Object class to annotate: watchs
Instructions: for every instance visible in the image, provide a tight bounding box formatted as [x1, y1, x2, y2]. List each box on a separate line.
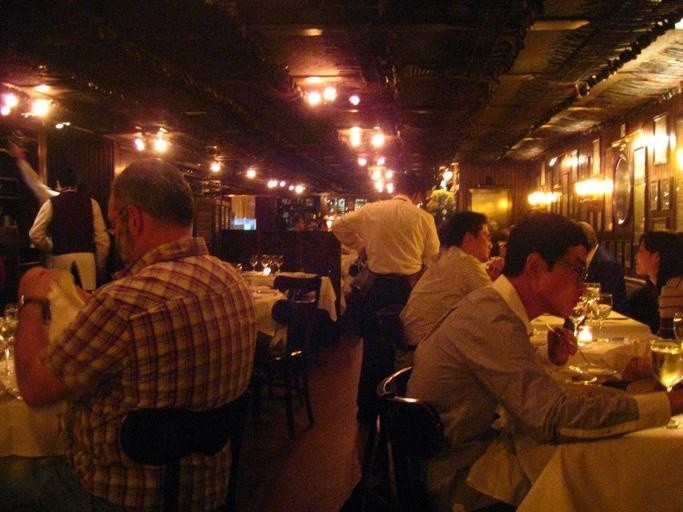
[15, 293, 46, 314]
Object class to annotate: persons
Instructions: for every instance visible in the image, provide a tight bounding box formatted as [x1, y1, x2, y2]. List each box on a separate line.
[28, 156, 110, 294]
[14, 156, 258, 511]
[332, 170, 442, 426]
[6, 139, 60, 204]
[400, 212, 683, 511]
[397, 207, 504, 379]
[574, 218, 627, 314]
[287, 210, 308, 231]
[622, 231, 683, 334]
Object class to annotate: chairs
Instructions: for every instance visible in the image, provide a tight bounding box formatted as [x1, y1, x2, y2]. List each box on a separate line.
[367, 304, 418, 454]
[121, 389, 251, 512]
[376, 364, 445, 509]
[254, 294, 320, 441]
[258, 275, 338, 408]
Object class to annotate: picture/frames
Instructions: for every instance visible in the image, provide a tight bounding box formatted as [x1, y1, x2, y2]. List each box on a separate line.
[540, 110, 674, 269]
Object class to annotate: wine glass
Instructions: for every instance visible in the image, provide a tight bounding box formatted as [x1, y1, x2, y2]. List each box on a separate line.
[557, 371, 597, 385]
[0, 304, 24, 377]
[647, 309, 682, 428]
[569, 281, 613, 348]
[547, 332, 570, 375]
[233, 254, 284, 275]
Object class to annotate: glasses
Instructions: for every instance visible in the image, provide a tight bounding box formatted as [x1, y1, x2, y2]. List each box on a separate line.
[562, 259, 590, 283]
